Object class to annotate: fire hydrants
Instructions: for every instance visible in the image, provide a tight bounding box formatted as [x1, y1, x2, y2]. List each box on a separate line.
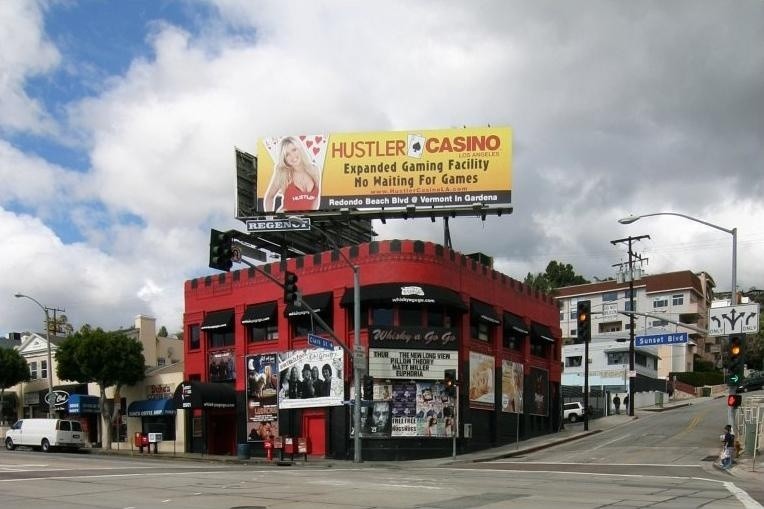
[265, 436, 274, 459]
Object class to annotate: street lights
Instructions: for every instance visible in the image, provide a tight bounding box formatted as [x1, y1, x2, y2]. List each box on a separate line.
[16, 295, 56, 418]
[620, 212, 737, 441]
[289, 217, 364, 463]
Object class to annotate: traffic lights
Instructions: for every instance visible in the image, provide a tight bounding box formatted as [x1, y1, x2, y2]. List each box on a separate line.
[284, 272, 297, 303]
[728, 394, 741, 406]
[728, 333, 745, 386]
[445, 369, 456, 392]
[210, 229, 233, 272]
[577, 300, 590, 341]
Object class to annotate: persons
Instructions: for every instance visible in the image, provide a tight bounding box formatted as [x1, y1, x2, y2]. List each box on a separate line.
[279, 363, 332, 399]
[613, 393, 620, 414]
[249, 422, 277, 440]
[623, 394, 629, 414]
[263, 137, 321, 212]
[382, 387, 390, 400]
[368, 401, 390, 432]
[721, 424, 737, 469]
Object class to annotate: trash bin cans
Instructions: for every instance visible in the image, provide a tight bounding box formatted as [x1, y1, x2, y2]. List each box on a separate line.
[744, 424, 760, 455]
[702, 388, 711, 396]
[655, 391, 663, 404]
[237, 444, 251, 460]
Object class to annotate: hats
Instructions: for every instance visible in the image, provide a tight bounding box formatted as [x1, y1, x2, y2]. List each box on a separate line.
[302, 363, 313, 372]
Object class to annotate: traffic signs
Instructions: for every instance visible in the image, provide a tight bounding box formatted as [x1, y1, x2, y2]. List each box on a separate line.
[708, 304, 760, 336]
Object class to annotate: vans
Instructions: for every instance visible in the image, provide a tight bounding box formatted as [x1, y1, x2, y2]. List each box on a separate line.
[563, 402, 583, 422]
[4, 418, 85, 452]
